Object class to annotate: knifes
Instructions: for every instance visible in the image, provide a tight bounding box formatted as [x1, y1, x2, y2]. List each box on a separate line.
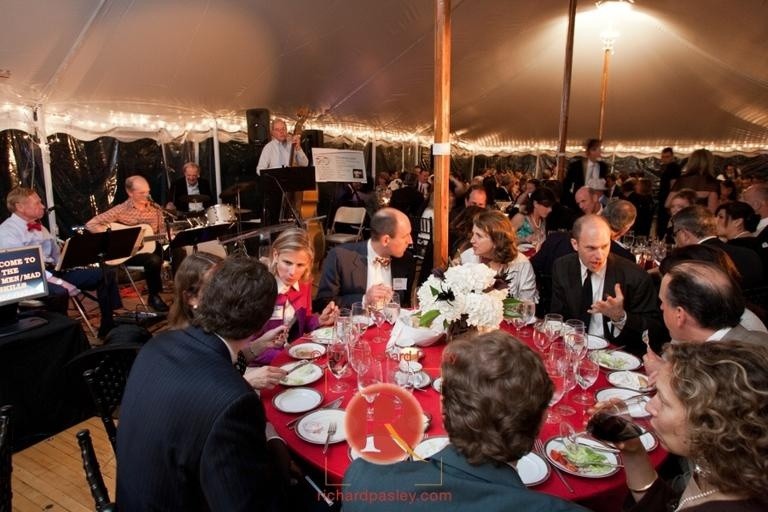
[288, 398, 346, 429]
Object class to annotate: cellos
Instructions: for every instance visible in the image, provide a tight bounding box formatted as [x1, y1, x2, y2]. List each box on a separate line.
[282, 107, 325, 263]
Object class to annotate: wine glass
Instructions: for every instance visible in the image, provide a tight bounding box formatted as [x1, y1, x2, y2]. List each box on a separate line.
[513, 227, 547, 259]
[587, 177, 606, 191]
[628, 232, 669, 270]
[509, 300, 599, 422]
[328, 290, 403, 428]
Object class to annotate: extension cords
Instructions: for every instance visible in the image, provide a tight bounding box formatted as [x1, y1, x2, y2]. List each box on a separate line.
[129, 310, 158, 318]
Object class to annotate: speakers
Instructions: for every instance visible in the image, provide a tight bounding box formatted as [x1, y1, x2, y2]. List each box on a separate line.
[300, 129, 323, 161]
[246, 108, 270, 147]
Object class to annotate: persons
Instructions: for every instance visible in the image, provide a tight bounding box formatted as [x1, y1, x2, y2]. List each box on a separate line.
[1, 118, 768, 510]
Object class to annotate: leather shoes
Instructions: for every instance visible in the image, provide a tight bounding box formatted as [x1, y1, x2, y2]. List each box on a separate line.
[147, 294, 169, 312]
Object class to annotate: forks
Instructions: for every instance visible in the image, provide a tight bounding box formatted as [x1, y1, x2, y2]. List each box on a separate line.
[319, 424, 336, 455]
[534, 438, 577, 493]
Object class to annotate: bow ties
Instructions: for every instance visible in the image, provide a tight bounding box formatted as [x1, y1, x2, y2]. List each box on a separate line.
[376, 258, 391, 267]
[27, 222, 42, 232]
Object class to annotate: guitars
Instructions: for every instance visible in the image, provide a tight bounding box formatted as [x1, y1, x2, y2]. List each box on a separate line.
[102, 222, 166, 267]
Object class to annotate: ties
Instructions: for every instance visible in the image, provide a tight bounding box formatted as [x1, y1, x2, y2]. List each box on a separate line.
[582, 269, 595, 334]
[589, 162, 596, 180]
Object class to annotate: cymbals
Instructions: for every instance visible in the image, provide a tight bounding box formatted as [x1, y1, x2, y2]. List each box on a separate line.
[188, 194, 207, 203]
[219, 183, 249, 200]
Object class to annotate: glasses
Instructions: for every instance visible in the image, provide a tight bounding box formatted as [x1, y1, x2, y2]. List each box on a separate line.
[273, 127, 287, 132]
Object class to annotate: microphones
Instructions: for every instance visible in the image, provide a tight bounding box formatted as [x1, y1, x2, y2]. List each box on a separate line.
[45, 204, 59, 213]
[146, 194, 155, 204]
[263, 171, 274, 179]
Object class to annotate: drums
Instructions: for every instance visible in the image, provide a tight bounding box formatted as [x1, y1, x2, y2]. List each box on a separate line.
[207, 203, 237, 227]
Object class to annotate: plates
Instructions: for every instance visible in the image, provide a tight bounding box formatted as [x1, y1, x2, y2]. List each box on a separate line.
[271, 326, 336, 414]
[296, 410, 350, 445]
[347, 443, 408, 465]
[408, 436, 550, 487]
[585, 331, 659, 419]
[392, 347, 444, 392]
[546, 419, 657, 479]
